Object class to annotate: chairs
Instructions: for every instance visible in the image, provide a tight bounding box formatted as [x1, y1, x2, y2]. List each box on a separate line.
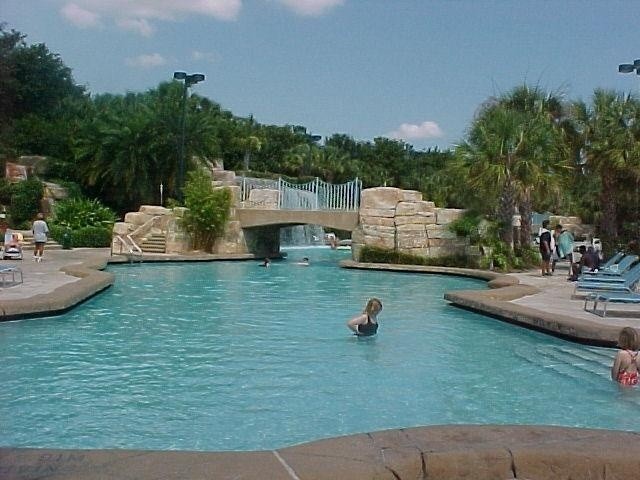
[0, 264, 23, 287]
[572, 251, 640, 318]
[3, 232, 25, 260]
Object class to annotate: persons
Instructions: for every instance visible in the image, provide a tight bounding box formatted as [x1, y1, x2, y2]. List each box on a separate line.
[612, 327, 640, 386]
[330, 236, 337, 250]
[346, 298, 382, 338]
[540, 219, 600, 281]
[5, 234, 22, 250]
[264, 258, 270, 268]
[32, 212, 49, 263]
[302, 257, 309, 262]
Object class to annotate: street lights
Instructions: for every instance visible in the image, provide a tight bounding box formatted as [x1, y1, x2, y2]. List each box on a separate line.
[173, 72, 206, 204]
